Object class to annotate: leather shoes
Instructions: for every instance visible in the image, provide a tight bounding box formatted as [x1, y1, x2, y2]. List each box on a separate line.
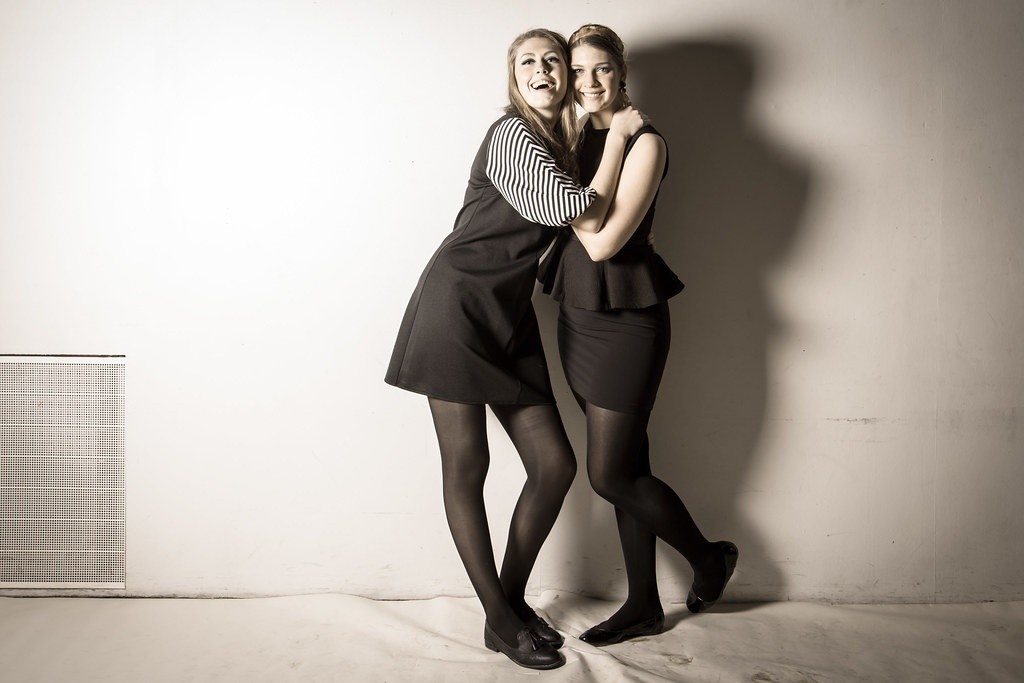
[579, 608, 665, 645]
[485, 617, 563, 670]
[686, 541, 739, 613]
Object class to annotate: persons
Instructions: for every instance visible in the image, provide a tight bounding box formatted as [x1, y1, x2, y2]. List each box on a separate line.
[541, 23, 741, 649]
[386, 27, 646, 671]
